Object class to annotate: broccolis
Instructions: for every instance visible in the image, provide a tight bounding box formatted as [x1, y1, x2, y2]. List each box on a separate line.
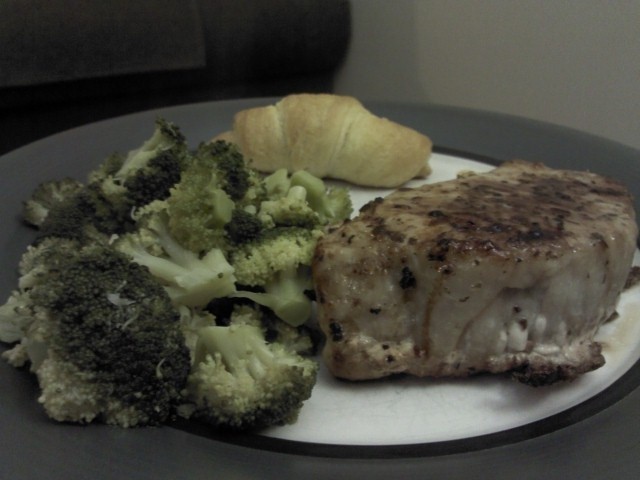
[0, 114, 353, 433]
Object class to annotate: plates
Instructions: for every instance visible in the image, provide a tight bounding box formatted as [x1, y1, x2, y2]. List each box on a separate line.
[0, 95, 640, 480]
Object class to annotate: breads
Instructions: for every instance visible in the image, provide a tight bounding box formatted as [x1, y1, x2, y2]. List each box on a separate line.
[214, 90, 433, 191]
